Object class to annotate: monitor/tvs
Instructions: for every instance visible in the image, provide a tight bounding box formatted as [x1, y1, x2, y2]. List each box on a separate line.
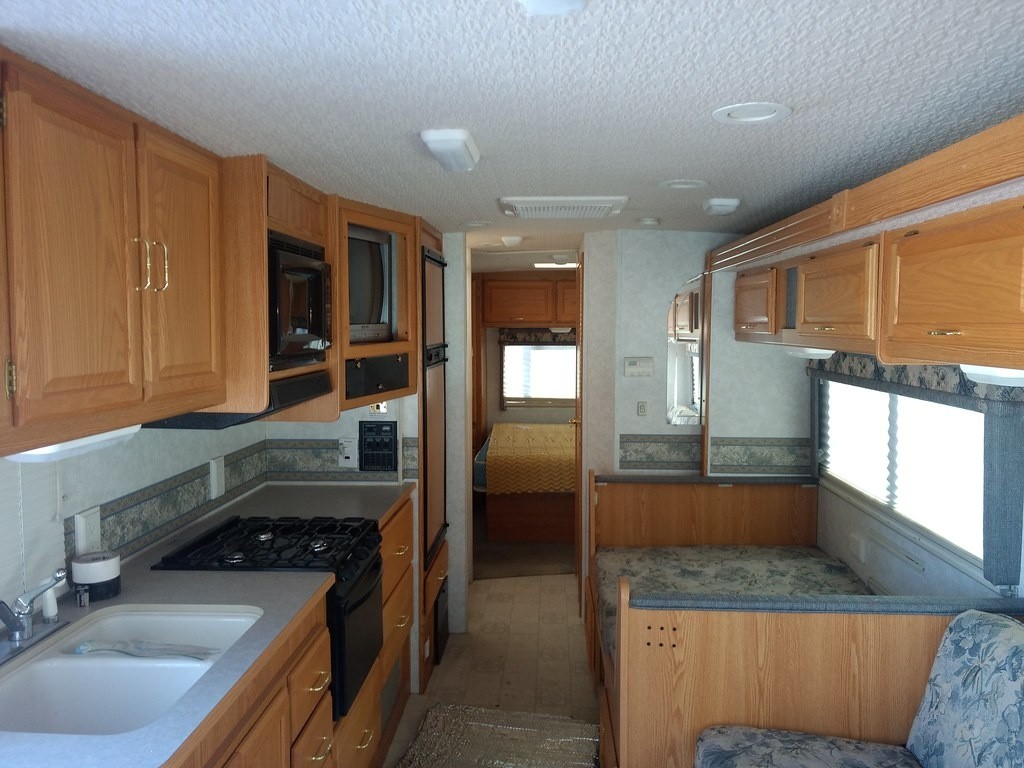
[346, 223, 394, 343]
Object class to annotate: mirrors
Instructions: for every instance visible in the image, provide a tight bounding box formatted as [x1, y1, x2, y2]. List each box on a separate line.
[667, 274, 703, 425]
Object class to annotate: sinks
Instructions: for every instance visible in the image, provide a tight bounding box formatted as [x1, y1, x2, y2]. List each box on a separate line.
[55, 605, 264, 660]
[0, 664, 215, 737]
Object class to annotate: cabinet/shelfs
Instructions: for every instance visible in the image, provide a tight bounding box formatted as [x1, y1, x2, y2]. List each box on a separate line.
[2, 482, 415, 767]
[0, 45, 226, 457]
[479, 272, 576, 327]
[668, 289, 700, 340]
[735, 195, 1023, 371]
[411, 216, 449, 696]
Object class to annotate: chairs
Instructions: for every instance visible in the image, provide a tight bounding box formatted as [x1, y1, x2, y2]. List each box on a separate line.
[694, 610, 1022, 767]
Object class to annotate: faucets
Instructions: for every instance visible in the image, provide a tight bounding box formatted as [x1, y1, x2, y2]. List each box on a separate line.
[0, 567, 67, 665]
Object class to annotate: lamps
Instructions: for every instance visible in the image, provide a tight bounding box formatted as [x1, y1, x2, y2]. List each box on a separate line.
[422, 130, 479, 176]
[703, 199, 740, 218]
[783, 345, 836, 359]
[958, 364, 1024, 387]
[549, 328, 572, 333]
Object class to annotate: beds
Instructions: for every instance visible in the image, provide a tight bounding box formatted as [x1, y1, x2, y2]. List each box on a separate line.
[586, 471, 1023, 768]
[473, 423, 575, 542]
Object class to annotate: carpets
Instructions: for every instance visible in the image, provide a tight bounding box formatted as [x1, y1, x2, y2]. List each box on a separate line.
[394, 705, 597, 767]
